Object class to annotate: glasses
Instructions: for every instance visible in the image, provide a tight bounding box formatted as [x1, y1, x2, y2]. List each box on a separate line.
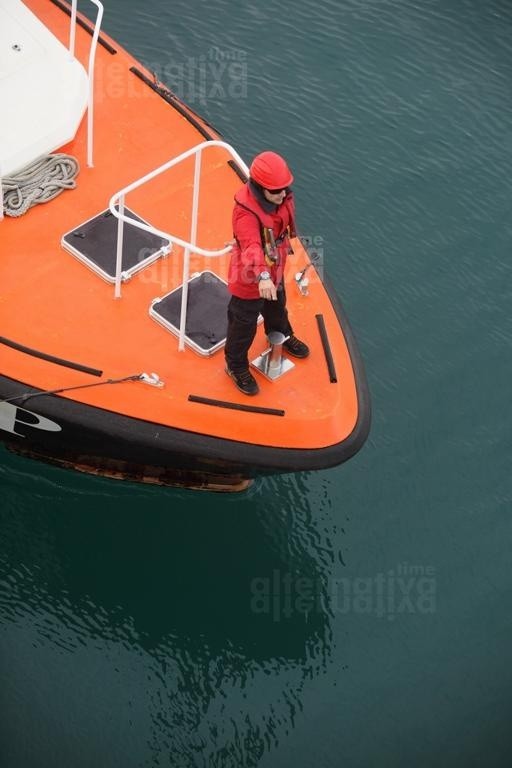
[262, 186, 288, 194]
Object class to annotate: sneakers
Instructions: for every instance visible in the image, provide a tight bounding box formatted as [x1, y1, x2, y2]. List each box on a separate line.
[226, 366, 258, 395]
[283, 335, 309, 357]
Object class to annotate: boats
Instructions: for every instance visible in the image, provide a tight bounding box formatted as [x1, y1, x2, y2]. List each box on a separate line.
[0, 0, 372, 489]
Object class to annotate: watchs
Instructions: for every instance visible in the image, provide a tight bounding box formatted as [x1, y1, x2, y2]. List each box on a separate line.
[254, 270, 272, 282]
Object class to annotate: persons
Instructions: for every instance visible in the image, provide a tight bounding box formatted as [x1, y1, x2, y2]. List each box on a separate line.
[223, 151, 311, 397]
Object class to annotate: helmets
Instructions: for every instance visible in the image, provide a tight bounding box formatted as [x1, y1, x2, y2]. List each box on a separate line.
[251, 151, 293, 190]
